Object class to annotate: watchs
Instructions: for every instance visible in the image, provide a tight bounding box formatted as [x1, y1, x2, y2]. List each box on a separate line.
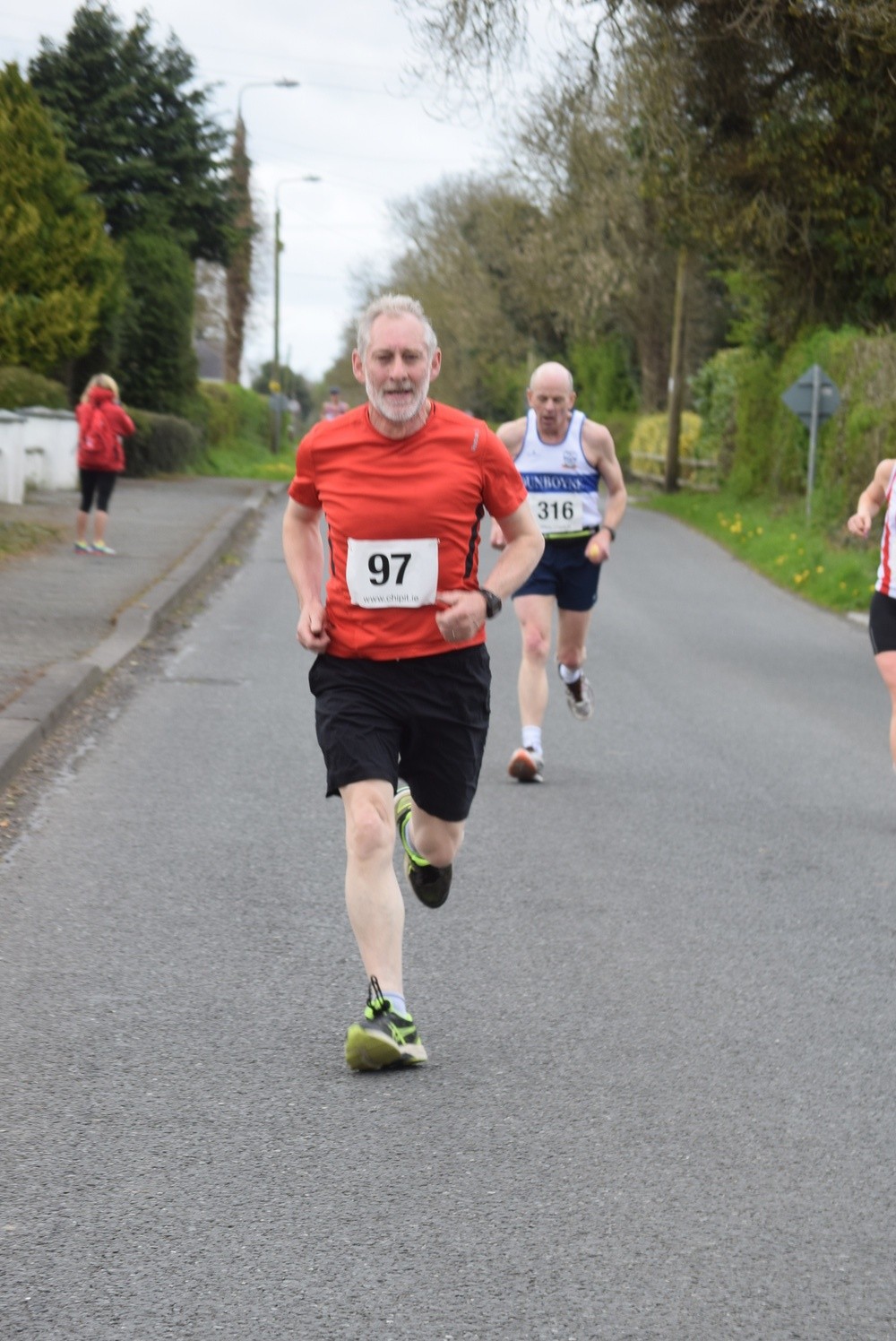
[479, 589, 502, 618]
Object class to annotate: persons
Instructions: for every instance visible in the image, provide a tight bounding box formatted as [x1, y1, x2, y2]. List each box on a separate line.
[492, 361, 628, 785]
[282, 292, 545, 1069]
[847, 458, 896, 762]
[320, 388, 348, 420]
[75, 373, 136, 554]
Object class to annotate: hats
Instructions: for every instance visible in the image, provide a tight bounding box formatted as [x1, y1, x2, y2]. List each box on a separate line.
[329, 388, 341, 395]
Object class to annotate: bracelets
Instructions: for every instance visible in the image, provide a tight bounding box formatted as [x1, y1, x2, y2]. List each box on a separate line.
[604, 524, 616, 540]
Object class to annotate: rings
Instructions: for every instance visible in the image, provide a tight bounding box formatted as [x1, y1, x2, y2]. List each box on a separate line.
[452, 631, 455, 638]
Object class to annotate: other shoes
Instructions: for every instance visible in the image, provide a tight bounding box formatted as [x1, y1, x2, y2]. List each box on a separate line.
[557, 663, 593, 720]
[507, 747, 545, 783]
[74, 543, 94, 556]
[91, 541, 115, 556]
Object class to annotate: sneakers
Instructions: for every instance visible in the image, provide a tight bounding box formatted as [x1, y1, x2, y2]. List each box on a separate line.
[346, 975, 428, 1072]
[393, 786, 453, 908]
